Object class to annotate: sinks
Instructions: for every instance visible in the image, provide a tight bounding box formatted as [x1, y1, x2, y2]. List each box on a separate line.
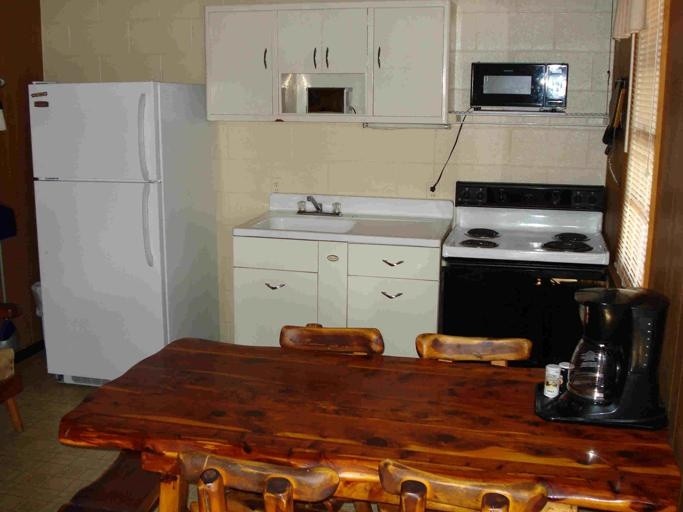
[253, 215, 359, 234]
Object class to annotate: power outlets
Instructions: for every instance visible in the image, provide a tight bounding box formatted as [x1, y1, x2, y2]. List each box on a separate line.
[271, 178, 282, 192]
[426, 182, 439, 199]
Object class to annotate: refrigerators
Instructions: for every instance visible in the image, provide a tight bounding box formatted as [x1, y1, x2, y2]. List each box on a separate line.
[29, 80, 219, 387]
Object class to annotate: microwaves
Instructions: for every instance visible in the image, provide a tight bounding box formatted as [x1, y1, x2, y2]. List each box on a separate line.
[469, 62, 569, 113]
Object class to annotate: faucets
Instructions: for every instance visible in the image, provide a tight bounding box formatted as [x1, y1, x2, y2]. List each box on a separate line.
[307, 195, 323, 213]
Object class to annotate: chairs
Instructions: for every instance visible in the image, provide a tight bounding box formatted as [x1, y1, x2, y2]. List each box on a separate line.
[0, 300, 24, 432]
[378, 457, 547, 512]
[278, 322, 384, 360]
[415, 333, 532, 368]
[179, 443, 340, 512]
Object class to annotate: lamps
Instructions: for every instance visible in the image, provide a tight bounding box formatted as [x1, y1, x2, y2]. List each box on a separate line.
[362, 122, 449, 129]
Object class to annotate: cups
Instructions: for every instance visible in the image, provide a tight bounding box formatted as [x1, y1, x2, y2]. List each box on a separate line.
[560, 361, 571, 395]
[544, 364, 564, 398]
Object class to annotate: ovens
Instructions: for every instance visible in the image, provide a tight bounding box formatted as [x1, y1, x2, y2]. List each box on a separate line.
[438, 263, 611, 368]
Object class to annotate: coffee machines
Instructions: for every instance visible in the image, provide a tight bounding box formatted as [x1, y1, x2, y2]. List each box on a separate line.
[535, 286, 669, 431]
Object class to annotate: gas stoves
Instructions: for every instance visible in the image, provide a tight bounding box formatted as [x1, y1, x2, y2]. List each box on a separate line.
[442, 226, 610, 255]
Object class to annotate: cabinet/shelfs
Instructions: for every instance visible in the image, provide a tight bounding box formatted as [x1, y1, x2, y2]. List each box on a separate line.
[233, 232, 319, 347]
[275, 1, 369, 124]
[204, 5, 275, 124]
[347, 242, 442, 358]
[370, 0, 451, 124]
